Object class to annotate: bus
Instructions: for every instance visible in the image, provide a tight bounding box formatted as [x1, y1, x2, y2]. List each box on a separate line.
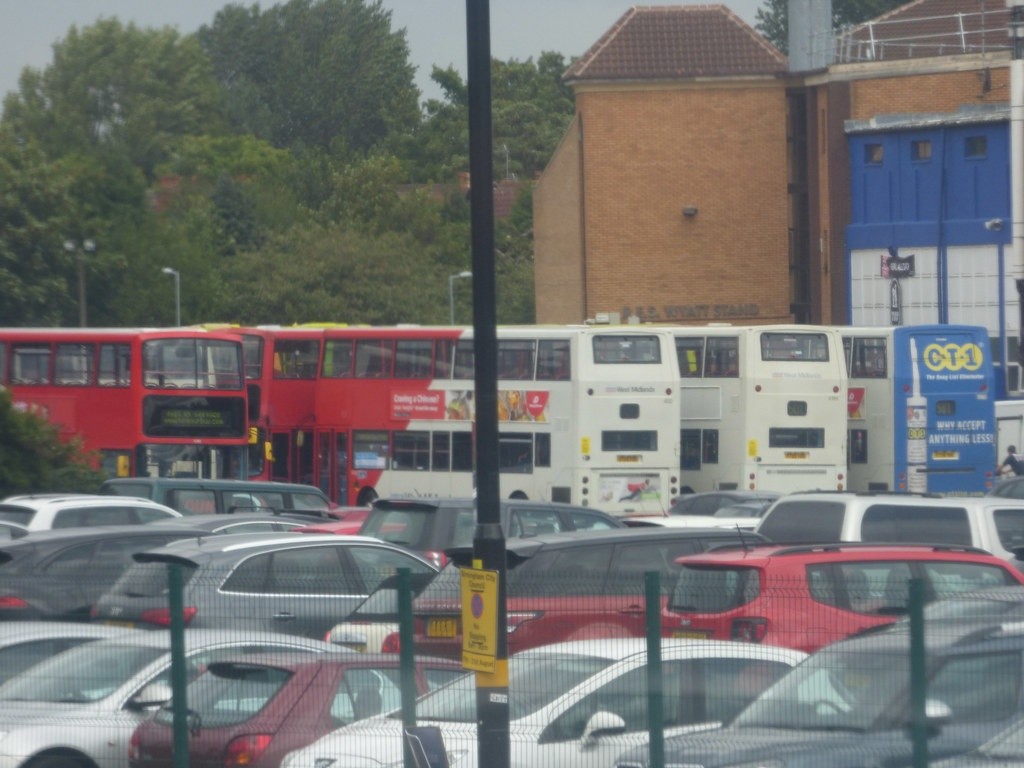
[1, 316, 1000, 517]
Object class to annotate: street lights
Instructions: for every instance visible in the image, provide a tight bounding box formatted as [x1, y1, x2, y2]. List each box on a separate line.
[160, 266, 182, 327]
[448, 270, 472, 326]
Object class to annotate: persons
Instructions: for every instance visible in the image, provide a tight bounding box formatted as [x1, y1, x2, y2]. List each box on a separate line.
[994, 445, 1024, 476]
[618, 479, 650, 503]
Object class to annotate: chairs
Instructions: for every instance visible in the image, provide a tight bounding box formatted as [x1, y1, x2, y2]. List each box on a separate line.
[355, 688, 382, 721]
[533, 524, 555, 537]
[274, 559, 307, 594]
[844, 569, 869, 604]
[770, 340, 826, 358]
[665, 543, 712, 583]
[336, 371, 571, 381]
[5, 378, 220, 390]
[882, 568, 913, 608]
[312, 557, 345, 593]
[962, 660, 1017, 723]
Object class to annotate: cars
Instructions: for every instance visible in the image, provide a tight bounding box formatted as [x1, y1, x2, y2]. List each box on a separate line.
[609, 606, 1024, 768]
[127, 650, 472, 768]
[1, 626, 447, 767]
[278, 635, 862, 768]
[0, 475, 1024, 620]
